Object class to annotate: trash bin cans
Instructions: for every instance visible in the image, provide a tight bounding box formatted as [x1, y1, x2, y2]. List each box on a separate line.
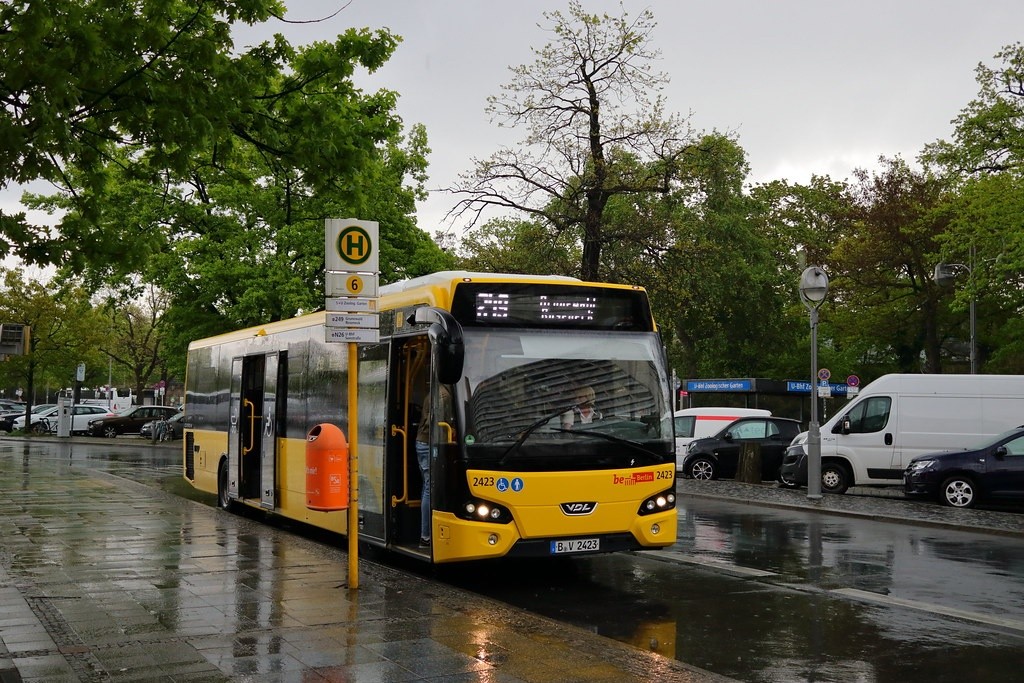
[734, 441, 761, 484]
[305, 423, 350, 511]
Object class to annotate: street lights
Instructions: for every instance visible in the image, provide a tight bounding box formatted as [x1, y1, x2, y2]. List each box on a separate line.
[797, 263, 838, 500]
[932, 261, 987, 378]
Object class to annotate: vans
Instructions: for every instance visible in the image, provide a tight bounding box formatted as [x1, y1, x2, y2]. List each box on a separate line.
[647, 406, 772, 474]
[787, 375, 1024, 495]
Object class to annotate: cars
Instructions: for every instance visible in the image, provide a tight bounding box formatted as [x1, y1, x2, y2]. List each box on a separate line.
[902, 427, 1024, 509]
[86, 404, 181, 439]
[682, 417, 803, 483]
[0, 399, 39, 433]
[141, 407, 185, 439]
[12, 404, 115, 434]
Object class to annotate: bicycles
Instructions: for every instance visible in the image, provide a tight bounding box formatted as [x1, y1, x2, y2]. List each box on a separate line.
[156, 416, 176, 442]
[30, 417, 58, 435]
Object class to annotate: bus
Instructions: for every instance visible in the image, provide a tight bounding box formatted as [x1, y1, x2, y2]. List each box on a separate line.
[54, 387, 133, 410]
[186, 270, 678, 567]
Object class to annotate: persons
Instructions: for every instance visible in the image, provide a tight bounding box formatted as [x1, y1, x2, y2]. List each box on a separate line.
[416, 383, 456, 548]
[562, 386, 602, 428]
